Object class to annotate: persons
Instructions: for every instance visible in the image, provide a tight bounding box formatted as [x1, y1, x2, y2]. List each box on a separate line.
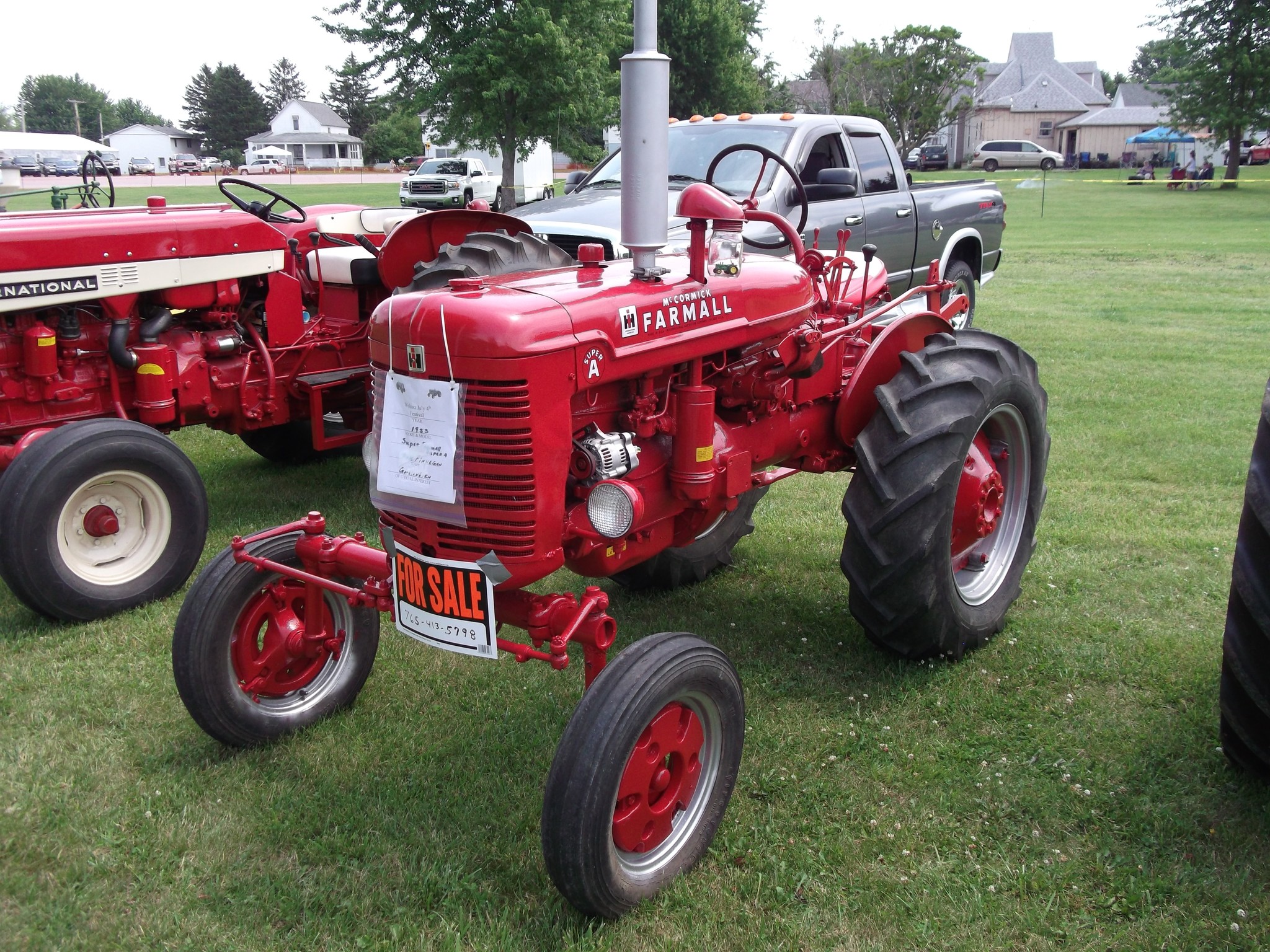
[1165, 162, 1187, 180]
[389, 158, 395, 173]
[1178, 150, 1198, 191]
[1195, 157, 1214, 180]
[1137, 160, 1153, 179]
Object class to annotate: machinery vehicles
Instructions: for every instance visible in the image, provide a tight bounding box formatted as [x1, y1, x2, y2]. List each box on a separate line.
[172, 0, 1052, 925]
[1, 178, 547, 626]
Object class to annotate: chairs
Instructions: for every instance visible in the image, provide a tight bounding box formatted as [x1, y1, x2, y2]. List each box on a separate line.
[1064, 150, 1175, 171]
[800, 152, 832, 185]
[1169, 173, 1215, 190]
[306, 208, 435, 284]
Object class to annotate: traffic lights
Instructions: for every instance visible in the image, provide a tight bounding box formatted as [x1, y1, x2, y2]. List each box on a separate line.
[427, 143, 430, 149]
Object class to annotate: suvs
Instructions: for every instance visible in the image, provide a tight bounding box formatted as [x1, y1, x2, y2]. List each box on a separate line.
[202, 156, 222, 163]
[12, 155, 42, 177]
[916, 144, 949, 172]
[128, 156, 156, 177]
[237, 159, 288, 176]
[168, 153, 202, 176]
[396, 156, 436, 172]
[971, 139, 1066, 172]
[36, 156, 62, 176]
[92, 152, 122, 176]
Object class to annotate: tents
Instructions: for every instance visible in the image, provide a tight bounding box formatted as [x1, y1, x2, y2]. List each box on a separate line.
[0, 131, 120, 179]
[252, 145, 295, 170]
[1118, 124, 1196, 180]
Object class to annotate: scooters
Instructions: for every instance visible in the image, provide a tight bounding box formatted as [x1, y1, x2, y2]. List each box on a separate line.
[222, 160, 233, 176]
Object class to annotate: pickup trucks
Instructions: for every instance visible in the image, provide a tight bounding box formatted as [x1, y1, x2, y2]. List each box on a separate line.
[400, 158, 503, 212]
[501, 111, 1008, 340]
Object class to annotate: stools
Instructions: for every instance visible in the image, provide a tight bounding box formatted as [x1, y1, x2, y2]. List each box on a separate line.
[780, 246, 889, 314]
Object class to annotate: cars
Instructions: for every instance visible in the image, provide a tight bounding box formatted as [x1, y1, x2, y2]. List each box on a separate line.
[77, 158, 93, 176]
[1246, 135, 1270, 165]
[55, 159, 79, 177]
[1220, 139, 1252, 166]
[199, 159, 222, 172]
[900, 147, 921, 170]
